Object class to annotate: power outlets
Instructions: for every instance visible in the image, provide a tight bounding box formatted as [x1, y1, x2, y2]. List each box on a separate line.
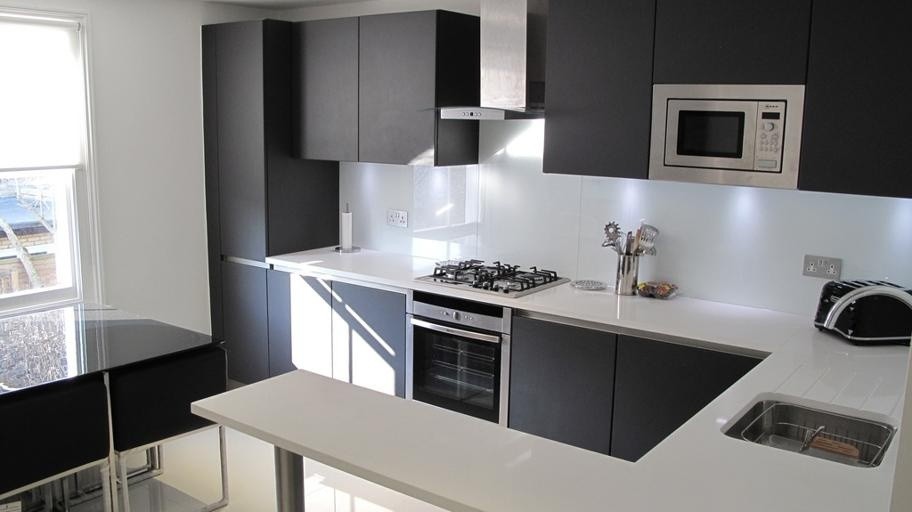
[803, 254, 842, 280]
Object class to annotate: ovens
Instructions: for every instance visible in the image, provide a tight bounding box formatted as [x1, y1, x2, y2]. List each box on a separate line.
[405, 315, 511, 427]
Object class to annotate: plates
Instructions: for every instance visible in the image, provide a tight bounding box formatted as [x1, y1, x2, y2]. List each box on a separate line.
[572, 279, 607, 292]
[638, 280, 679, 299]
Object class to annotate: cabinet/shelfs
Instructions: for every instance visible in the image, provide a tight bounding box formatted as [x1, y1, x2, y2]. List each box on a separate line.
[509, 313, 764, 460]
[797, 1, 911, 198]
[652, 0, 811, 84]
[291, 10, 479, 167]
[543, 0, 655, 180]
[267, 269, 405, 399]
[202, 20, 339, 384]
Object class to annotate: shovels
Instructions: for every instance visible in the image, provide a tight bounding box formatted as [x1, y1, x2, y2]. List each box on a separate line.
[638, 225, 659, 256]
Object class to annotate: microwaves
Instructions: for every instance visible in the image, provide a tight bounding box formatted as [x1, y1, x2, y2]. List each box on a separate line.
[663, 99, 785, 173]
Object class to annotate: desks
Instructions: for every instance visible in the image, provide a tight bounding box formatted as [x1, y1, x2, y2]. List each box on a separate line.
[1, 297, 229, 512]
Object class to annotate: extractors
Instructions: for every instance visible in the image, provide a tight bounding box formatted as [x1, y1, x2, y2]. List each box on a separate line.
[438, 2, 544, 121]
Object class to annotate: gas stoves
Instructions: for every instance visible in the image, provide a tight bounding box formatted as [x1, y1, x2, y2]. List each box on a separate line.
[417, 258, 560, 295]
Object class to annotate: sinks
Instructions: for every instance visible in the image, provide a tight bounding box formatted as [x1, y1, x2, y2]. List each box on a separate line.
[720, 391, 896, 468]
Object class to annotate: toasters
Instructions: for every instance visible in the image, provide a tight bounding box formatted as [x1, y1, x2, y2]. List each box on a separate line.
[815, 276, 912, 345]
